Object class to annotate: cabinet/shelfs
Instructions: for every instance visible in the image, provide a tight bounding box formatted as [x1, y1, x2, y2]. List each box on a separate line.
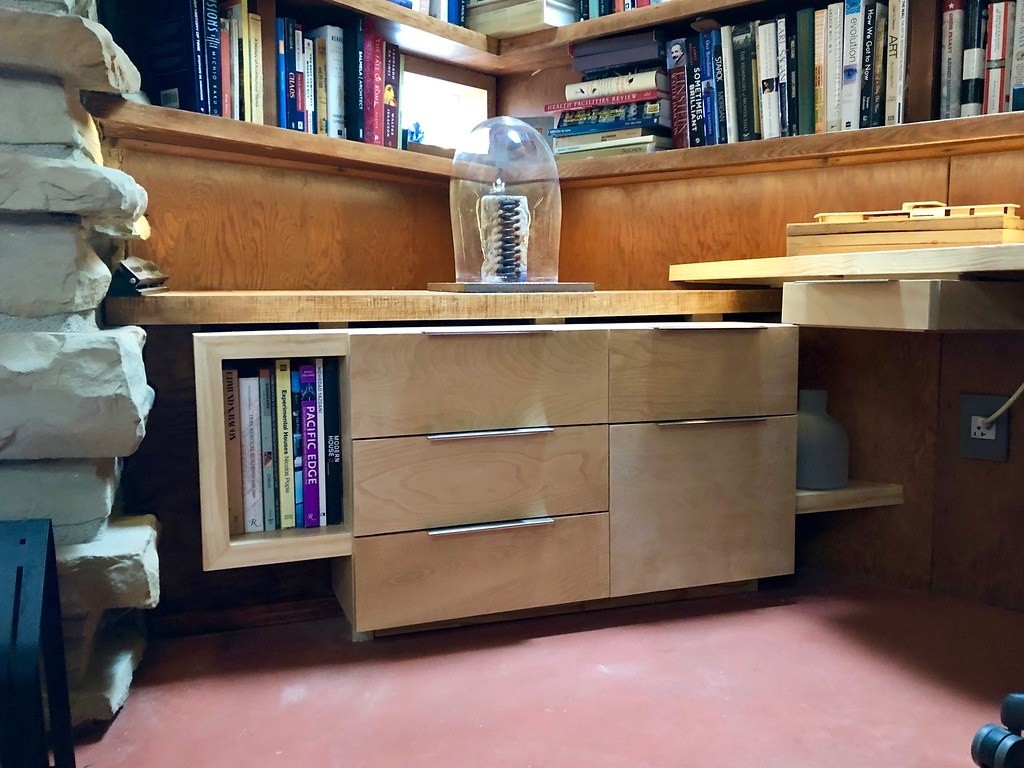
[193, 324, 906, 642]
[88, 0, 1024, 639]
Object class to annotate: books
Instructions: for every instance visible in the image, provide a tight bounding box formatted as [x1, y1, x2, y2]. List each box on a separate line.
[386, 0, 465, 29]
[493, 28, 674, 170]
[665, 0, 1024, 148]
[96, 0, 408, 152]
[222, 358, 345, 536]
[465, 0, 667, 38]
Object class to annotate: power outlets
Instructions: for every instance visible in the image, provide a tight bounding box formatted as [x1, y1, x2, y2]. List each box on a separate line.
[958, 394, 1008, 462]
